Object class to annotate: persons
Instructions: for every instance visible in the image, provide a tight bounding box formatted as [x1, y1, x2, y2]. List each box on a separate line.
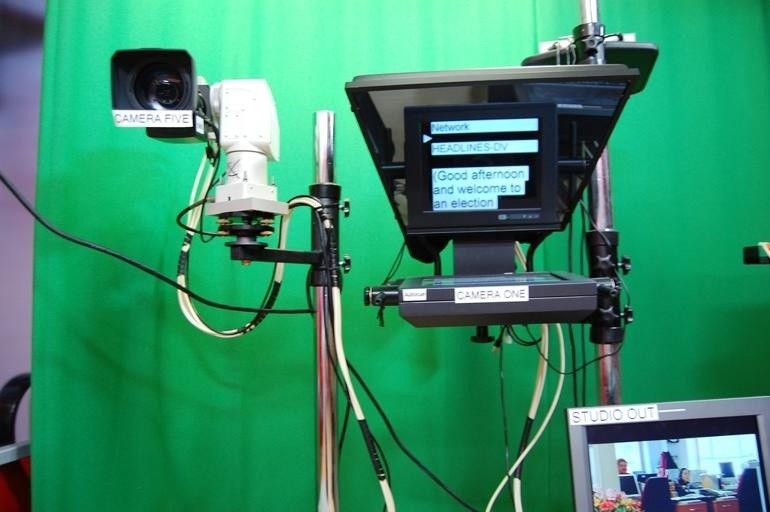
[676, 467, 696, 495]
[617, 459, 630, 474]
[658, 451, 678, 477]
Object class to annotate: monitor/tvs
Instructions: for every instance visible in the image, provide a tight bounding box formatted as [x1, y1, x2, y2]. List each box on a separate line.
[619, 473, 642, 498]
[665, 468, 681, 482]
[688, 469, 708, 489]
[719, 462, 735, 478]
[638, 473, 657, 483]
